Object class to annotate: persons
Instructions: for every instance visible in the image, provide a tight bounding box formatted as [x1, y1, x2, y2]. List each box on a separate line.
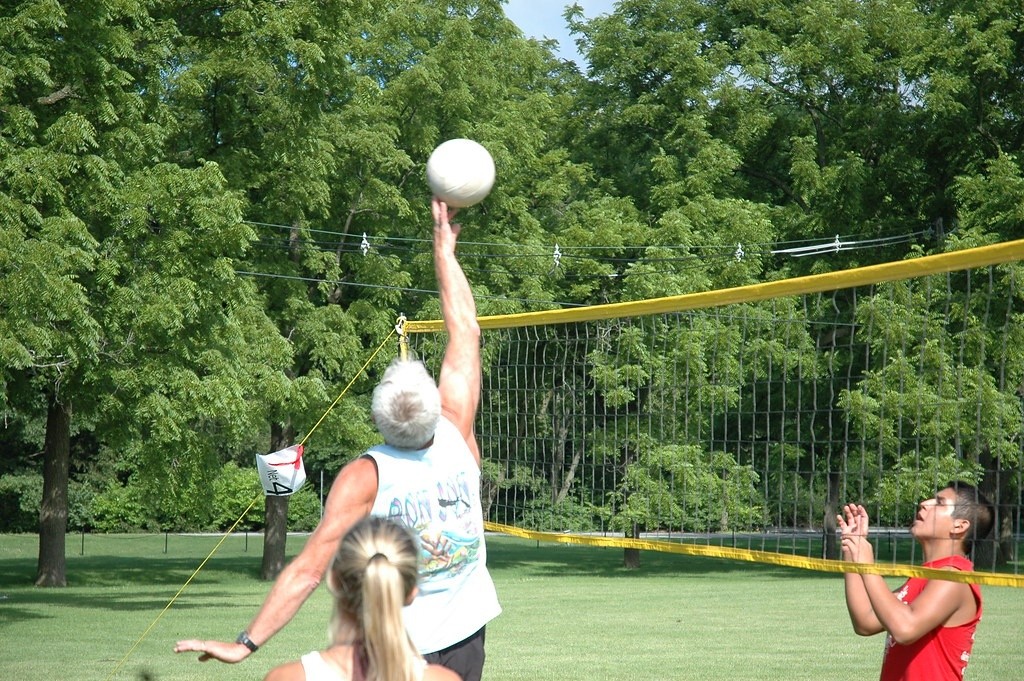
[836, 481, 995, 681]
[173, 194, 501, 681]
[263, 518, 465, 681]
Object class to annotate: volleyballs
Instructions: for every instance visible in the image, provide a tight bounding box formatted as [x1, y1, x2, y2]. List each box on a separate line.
[425, 138, 497, 209]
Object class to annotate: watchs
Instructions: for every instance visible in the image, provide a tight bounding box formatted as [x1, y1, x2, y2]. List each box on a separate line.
[237, 631, 258, 652]
